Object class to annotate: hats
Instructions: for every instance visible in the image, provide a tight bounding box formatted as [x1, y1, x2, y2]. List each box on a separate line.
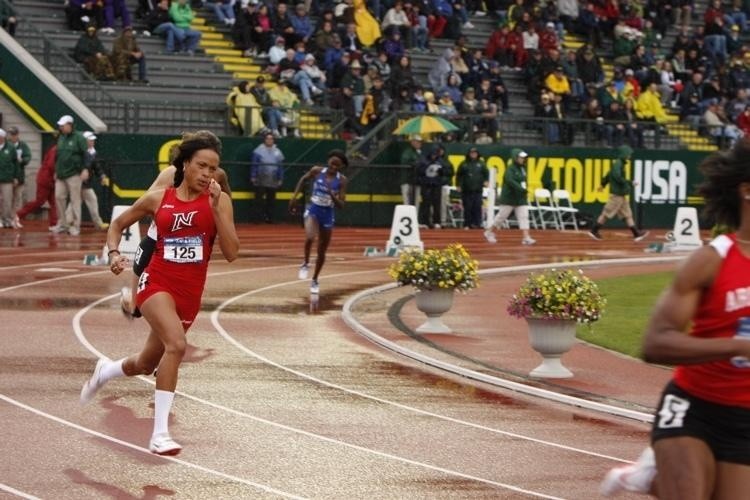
[83, 130, 97, 141]
[57, 115, 75, 126]
[0, 126, 20, 137]
[517, 151, 528, 157]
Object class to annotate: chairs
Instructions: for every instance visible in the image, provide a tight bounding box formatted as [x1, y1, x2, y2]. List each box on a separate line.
[432, 185, 581, 233]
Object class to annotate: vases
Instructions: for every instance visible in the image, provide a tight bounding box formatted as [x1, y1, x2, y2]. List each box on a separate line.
[413, 285, 454, 335]
[525, 317, 578, 377]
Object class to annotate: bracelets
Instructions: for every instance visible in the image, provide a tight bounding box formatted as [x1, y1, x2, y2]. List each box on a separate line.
[108, 248, 120, 257]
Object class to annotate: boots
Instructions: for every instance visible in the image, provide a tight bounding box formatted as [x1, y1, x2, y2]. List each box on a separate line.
[588, 221, 604, 240]
[628, 223, 649, 242]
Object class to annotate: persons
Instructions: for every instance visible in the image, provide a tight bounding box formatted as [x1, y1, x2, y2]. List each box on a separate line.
[483, 149, 536, 246]
[248, 135, 286, 224]
[401, 136, 488, 231]
[121, 131, 241, 381]
[594, 129, 749, 500]
[79, 128, 241, 456]
[3, 112, 113, 240]
[587, 142, 651, 242]
[287, 148, 350, 295]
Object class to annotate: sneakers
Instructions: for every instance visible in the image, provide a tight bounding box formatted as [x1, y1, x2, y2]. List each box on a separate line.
[521, 237, 536, 245]
[147, 429, 184, 456]
[484, 228, 498, 244]
[310, 278, 320, 294]
[68, 226, 80, 235]
[80, 355, 114, 402]
[298, 263, 309, 280]
[48, 224, 68, 232]
[601, 447, 658, 497]
[94, 223, 109, 231]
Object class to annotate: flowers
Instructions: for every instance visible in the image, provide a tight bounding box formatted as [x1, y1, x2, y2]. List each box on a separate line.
[386, 242, 484, 297]
[505, 266, 609, 334]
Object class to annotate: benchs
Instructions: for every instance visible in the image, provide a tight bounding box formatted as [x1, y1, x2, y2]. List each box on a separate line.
[1, 1, 335, 137]
[410, 2, 721, 150]
